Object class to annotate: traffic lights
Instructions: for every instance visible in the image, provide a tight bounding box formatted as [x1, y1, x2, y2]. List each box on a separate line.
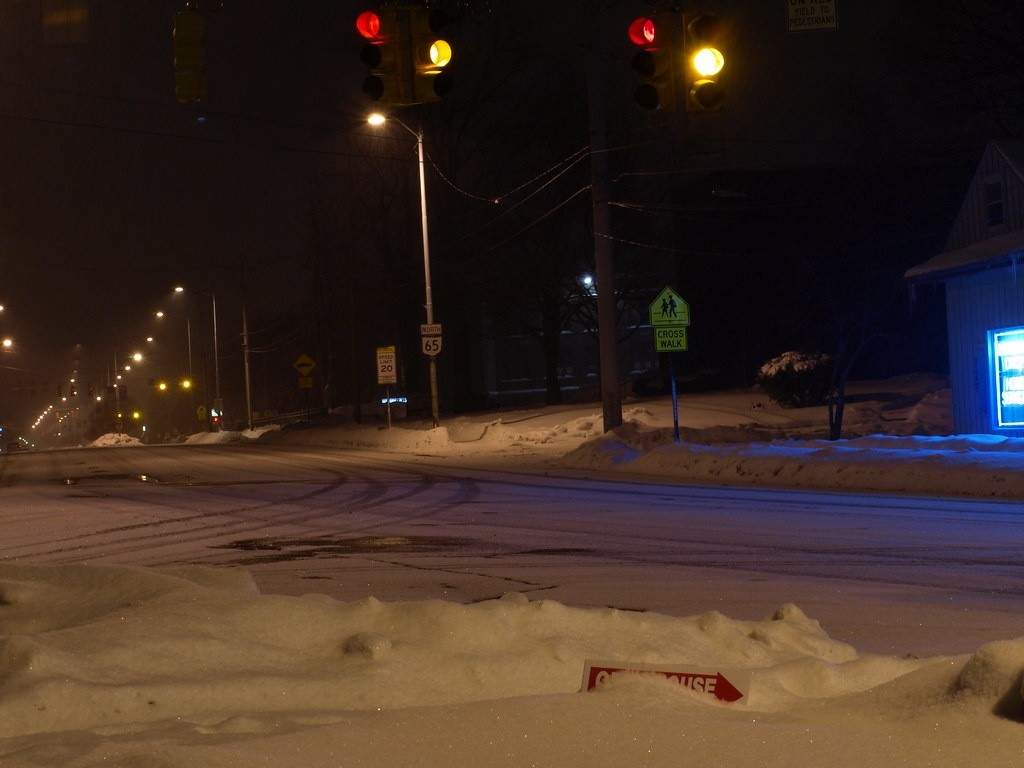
[355, 3, 410, 107]
[407, 5, 455, 103]
[213, 416, 220, 423]
[627, 9, 677, 116]
[681, 4, 727, 114]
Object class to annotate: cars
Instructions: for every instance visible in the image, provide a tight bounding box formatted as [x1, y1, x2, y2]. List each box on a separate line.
[6, 442, 29, 452]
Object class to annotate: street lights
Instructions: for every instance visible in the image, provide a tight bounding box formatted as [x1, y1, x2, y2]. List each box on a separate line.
[174, 283, 221, 433]
[366, 110, 440, 429]
[32, 309, 195, 447]
